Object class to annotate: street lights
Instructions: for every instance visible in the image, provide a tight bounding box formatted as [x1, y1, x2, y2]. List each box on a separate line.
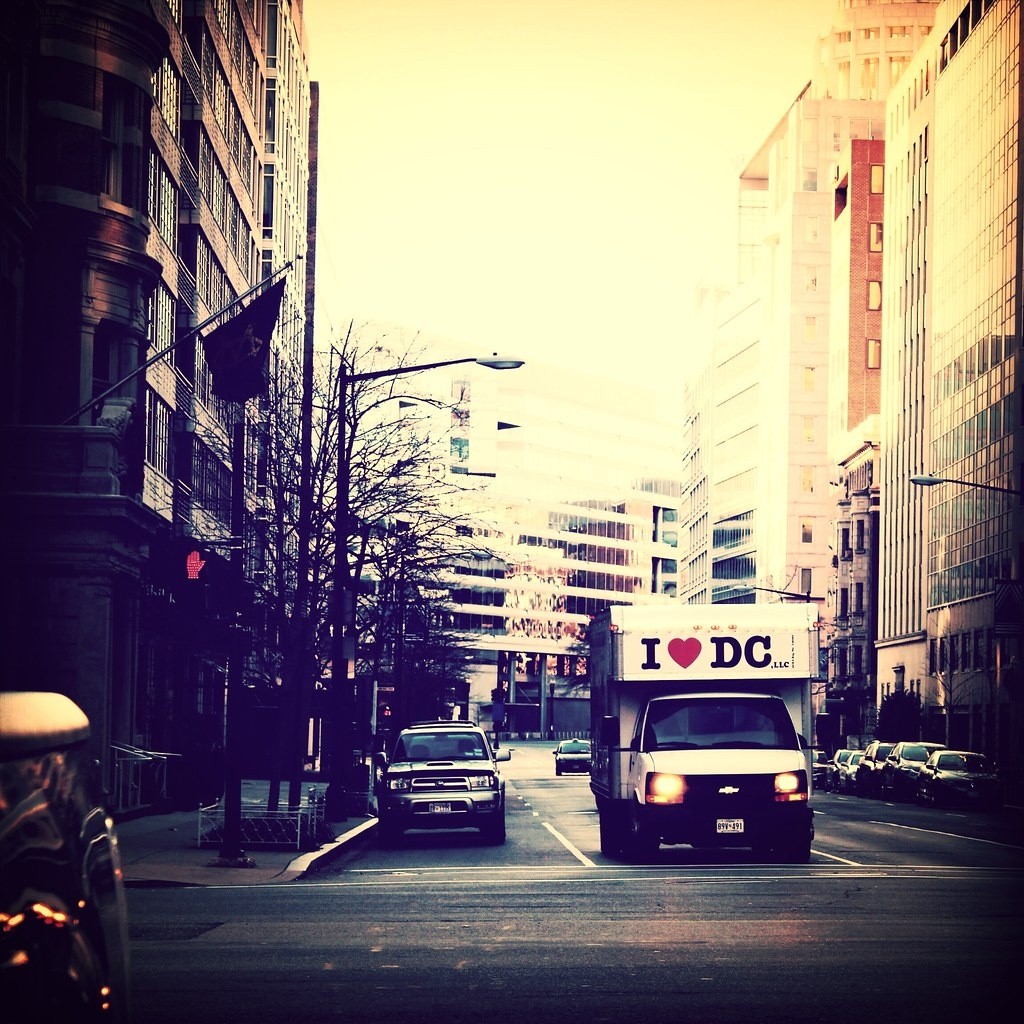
[909, 474, 1024, 498]
[391, 550, 494, 754]
[329, 352, 526, 821]
[733, 585, 812, 603]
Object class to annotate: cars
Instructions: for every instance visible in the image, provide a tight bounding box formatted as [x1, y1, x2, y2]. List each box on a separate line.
[839, 753, 866, 794]
[855, 743, 897, 799]
[826, 749, 866, 792]
[553, 739, 592, 776]
[881, 741, 949, 802]
[812, 750, 835, 790]
[916, 750, 1004, 813]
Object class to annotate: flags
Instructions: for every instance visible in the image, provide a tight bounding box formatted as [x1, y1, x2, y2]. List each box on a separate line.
[201, 274, 287, 406]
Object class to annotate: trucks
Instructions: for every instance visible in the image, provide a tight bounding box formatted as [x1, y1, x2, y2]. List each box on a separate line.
[588, 603, 820, 862]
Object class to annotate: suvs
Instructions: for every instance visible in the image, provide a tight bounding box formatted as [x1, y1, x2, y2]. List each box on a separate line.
[378, 719, 511, 848]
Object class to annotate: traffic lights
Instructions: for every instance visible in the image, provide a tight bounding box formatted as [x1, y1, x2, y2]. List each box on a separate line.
[491, 689, 503, 703]
[549, 683, 555, 693]
